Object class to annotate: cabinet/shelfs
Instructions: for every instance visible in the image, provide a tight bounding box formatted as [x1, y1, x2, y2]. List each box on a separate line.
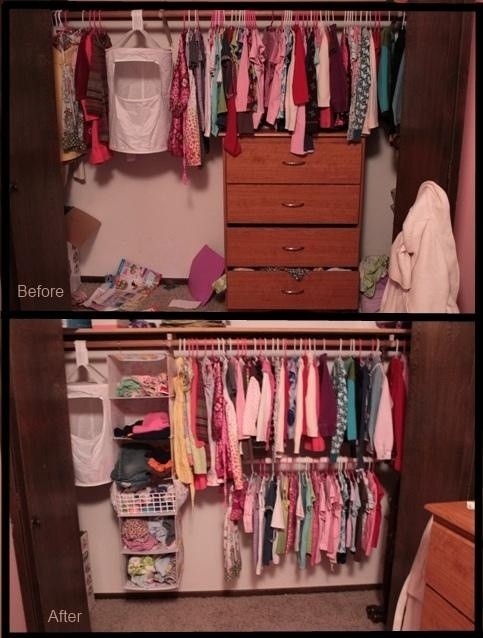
[216, 130, 367, 311]
[415, 500, 475, 632]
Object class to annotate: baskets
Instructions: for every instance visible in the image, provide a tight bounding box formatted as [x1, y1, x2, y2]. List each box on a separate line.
[114, 493, 176, 514]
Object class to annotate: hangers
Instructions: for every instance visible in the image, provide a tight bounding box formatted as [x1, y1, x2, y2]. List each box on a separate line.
[174, 337, 404, 382]
[227, 456, 383, 511]
